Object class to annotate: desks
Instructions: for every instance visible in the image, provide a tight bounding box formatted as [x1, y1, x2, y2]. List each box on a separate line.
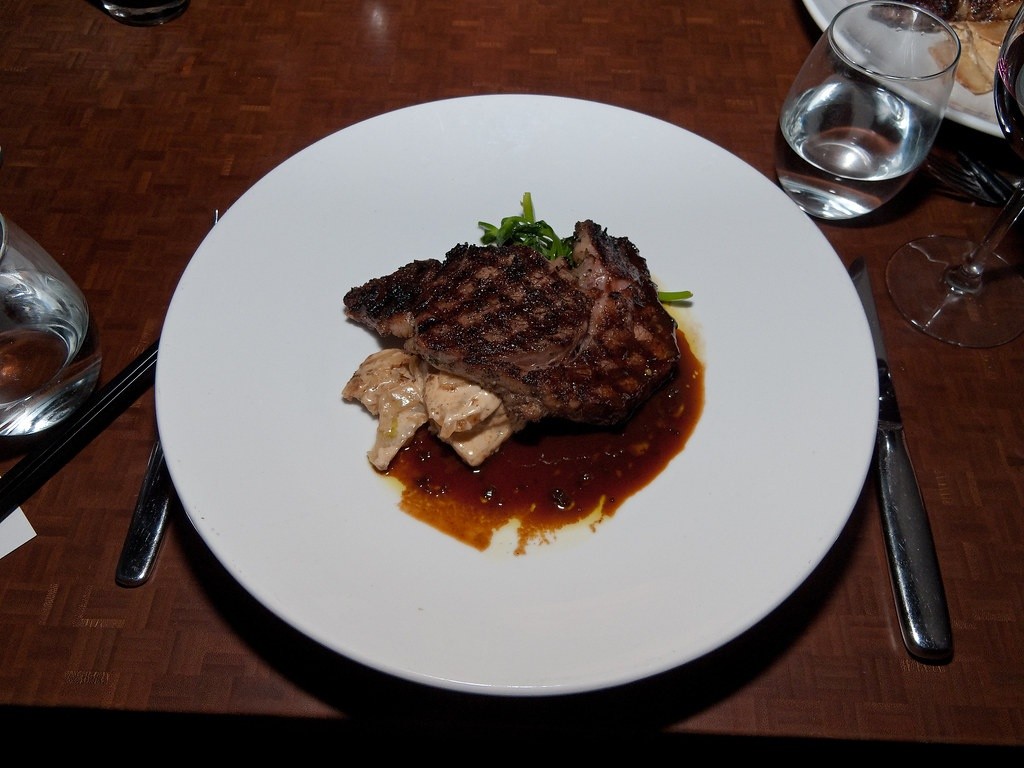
[0, 0, 1024, 768]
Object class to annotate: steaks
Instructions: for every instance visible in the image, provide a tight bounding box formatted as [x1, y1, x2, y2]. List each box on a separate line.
[342, 219, 680, 425]
[867, 0, 1024, 33]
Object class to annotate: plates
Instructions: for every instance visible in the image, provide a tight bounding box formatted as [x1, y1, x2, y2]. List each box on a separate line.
[801, 0, 1024, 142]
[155, 92, 880, 698]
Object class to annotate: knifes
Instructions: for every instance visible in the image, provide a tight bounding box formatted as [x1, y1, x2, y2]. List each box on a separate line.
[846, 256, 954, 662]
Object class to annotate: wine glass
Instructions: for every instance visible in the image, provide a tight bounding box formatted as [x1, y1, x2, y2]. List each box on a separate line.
[887, 4, 1024, 348]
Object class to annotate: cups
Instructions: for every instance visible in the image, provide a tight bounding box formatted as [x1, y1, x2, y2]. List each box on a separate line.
[774, 0, 962, 221]
[82, 0, 191, 26]
[0, 213, 102, 437]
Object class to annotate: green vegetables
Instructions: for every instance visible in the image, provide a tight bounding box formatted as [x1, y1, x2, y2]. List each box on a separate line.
[476, 193, 694, 301]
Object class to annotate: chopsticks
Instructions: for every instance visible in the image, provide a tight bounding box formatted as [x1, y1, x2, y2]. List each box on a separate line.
[0, 339, 162, 524]
